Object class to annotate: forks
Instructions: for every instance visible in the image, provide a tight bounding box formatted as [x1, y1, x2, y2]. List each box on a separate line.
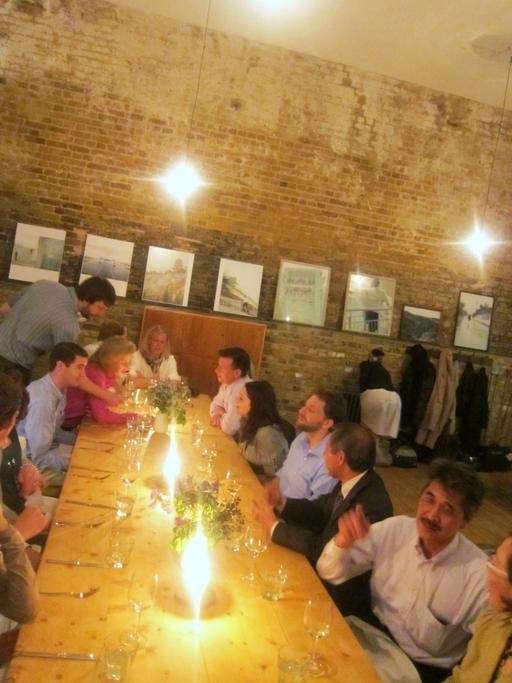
[53, 517, 110, 530]
[69, 423, 118, 510]
[34, 584, 95, 604]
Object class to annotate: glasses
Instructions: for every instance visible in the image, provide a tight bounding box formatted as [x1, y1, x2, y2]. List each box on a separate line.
[486, 558, 508, 578]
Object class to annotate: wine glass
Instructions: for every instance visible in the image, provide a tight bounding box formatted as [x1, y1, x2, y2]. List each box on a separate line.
[181, 404, 328, 674]
[96, 373, 158, 680]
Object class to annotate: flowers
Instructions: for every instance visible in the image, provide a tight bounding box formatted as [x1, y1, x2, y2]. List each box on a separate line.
[149, 474, 244, 549]
[143, 375, 195, 424]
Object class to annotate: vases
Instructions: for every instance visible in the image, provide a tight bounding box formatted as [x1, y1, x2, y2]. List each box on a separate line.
[150, 409, 172, 434]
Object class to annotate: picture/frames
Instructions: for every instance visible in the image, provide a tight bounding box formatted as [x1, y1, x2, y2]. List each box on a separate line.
[271, 260, 496, 351]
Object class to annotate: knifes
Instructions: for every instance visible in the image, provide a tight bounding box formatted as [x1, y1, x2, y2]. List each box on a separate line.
[14, 650, 92, 660]
[45, 556, 98, 570]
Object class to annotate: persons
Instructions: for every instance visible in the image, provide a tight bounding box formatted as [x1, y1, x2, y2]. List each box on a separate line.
[442, 530, 511, 683]
[235, 380, 297, 483]
[1, 275, 117, 386]
[1, 318, 183, 683]
[251, 420, 394, 566]
[243, 294, 249, 313]
[208, 346, 254, 443]
[314, 458, 491, 682]
[364, 278, 390, 331]
[359, 350, 396, 390]
[265, 388, 348, 501]
